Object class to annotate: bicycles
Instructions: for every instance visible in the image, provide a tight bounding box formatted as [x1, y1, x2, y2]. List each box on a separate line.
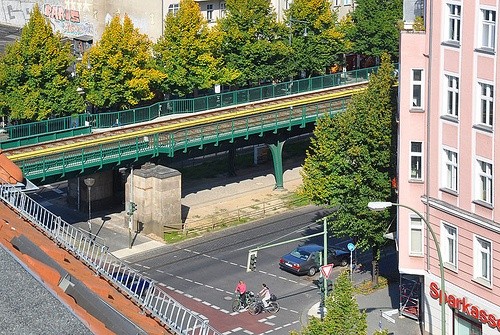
[248, 293, 280, 316]
[232, 290, 256, 312]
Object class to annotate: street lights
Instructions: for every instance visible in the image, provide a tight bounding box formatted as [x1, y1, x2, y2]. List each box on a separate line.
[367, 202, 446, 335]
[288, 14, 310, 49]
[119, 166, 133, 249]
[84, 178, 94, 246]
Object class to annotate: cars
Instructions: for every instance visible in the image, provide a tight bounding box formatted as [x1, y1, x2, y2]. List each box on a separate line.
[107, 271, 154, 297]
[278, 243, 353, 277]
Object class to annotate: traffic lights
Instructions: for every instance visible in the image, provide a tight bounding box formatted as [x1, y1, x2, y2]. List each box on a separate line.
[130, 201, 137, 212]
[247, 251, 257, 272]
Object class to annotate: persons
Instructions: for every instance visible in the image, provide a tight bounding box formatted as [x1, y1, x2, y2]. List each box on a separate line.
[234, 280, 246, 307]
[257, 284, 270, 309]
